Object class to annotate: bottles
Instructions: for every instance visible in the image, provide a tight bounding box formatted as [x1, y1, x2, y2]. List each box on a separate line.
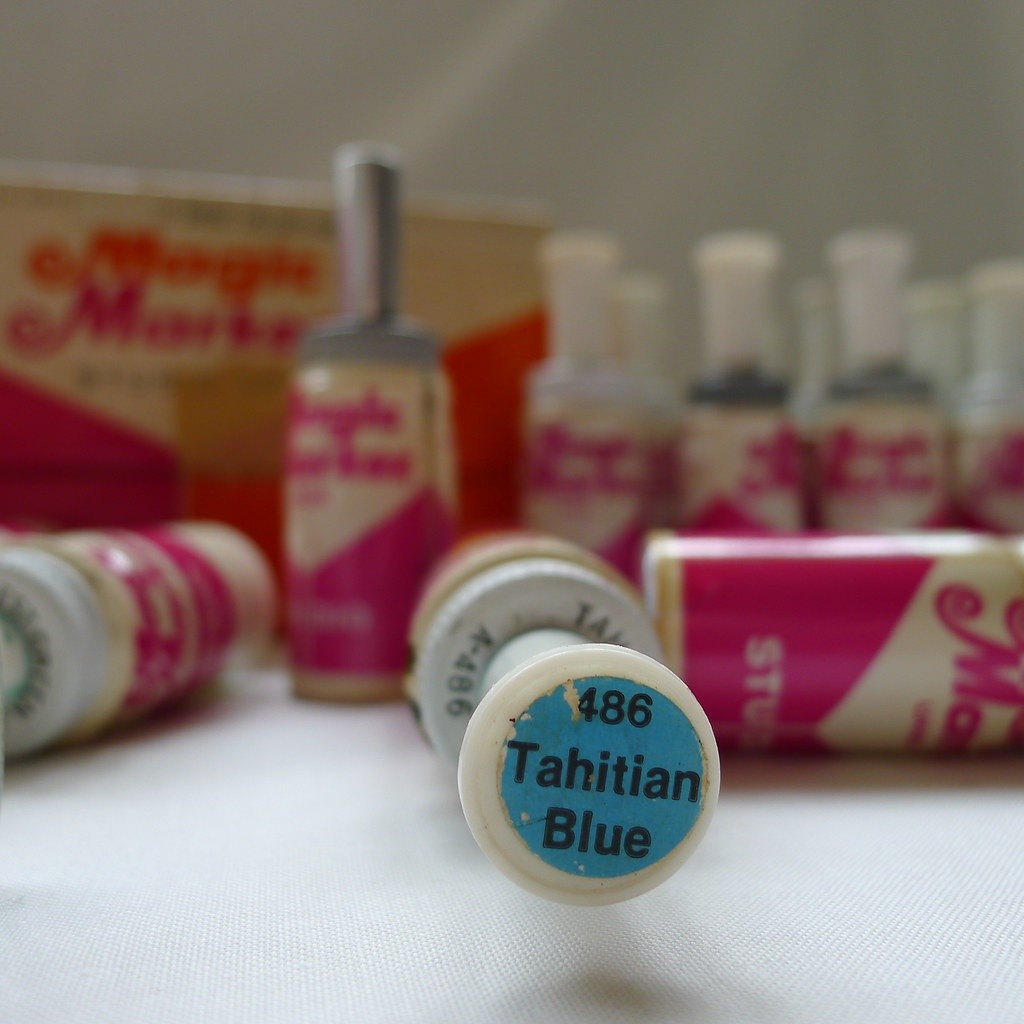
[1, 519, 275, 753]
[284, 157, 456, 695]
[525, 230, 1023, 757]
